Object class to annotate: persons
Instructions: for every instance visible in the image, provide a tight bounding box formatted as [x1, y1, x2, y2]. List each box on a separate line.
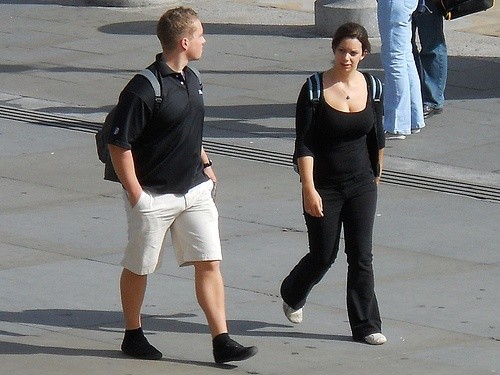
[280, 22, 388, 345]
[375, 0, 448, 140]
[105, 6, 260, 365]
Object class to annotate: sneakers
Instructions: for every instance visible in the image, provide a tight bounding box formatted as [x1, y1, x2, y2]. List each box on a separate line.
[212, 333, 257, 364]
[121, 328, 163, 359]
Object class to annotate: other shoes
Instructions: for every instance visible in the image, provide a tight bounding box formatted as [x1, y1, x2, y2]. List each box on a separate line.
[410, 129, 421, 134]
[423, 104, 444, 118]
[365, 333, 386, 345]
[383, 131, 406, 140]
[283, 300, 303, 324]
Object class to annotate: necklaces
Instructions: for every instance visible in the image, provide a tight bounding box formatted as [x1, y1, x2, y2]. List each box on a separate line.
[338, 79, 353, 100]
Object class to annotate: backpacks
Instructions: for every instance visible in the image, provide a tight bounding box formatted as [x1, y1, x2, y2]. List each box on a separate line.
[95, 66, 203, 183]
[438, 0, 494, 20]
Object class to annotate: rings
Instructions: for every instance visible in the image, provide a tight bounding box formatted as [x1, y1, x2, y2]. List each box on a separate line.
[308, 209, 311, 211]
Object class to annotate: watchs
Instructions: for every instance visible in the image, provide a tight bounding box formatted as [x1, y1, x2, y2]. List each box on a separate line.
[204, 159, 213, 168]
